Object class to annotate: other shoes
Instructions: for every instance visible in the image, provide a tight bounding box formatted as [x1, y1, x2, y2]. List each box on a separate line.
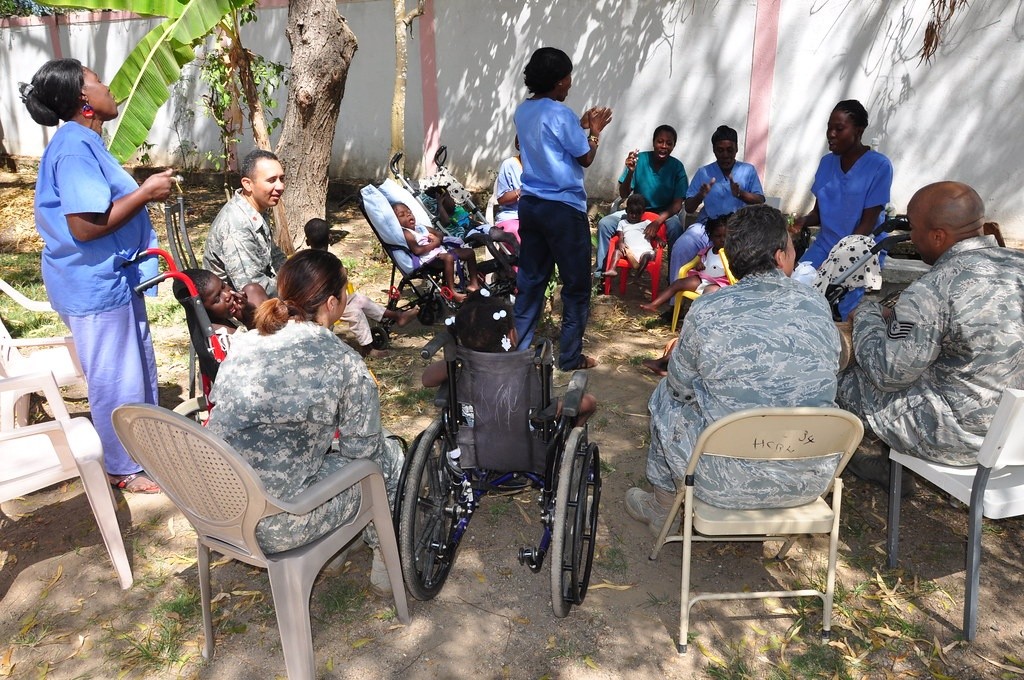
[596, 280, 605, 293]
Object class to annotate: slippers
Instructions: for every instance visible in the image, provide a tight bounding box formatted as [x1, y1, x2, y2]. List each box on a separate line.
[107, 471, 160, 494]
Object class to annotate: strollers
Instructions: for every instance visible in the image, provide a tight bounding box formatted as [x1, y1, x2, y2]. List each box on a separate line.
[120, 248, 409, 454]
[355, 143, 522, 350]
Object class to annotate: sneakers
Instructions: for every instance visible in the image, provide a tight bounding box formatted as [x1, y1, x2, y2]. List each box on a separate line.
[320, 535, 365, 578]
[370, 556, 393, 598]
[847, 447, 915, 498]
[624, 487, 681, 539]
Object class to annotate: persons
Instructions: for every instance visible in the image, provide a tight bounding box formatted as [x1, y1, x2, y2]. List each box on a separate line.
[838, 181, 1023, 497]
[513, 48, 612, 370]
[175, 99, 891, 377]
[200, 250, 408, 598]
[19, 57, 172, 495]
[420, 288, 597, 431]
[626, 206, 841, 539]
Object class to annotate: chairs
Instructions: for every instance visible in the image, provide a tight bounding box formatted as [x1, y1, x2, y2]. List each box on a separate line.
[110, 396, 410, 680]
[648, 407, 865, 654]
[605, 194, 703, 333]
[164, 196, 201, 401]
[0, 276, 133, 591]
[889, 389, 1024, 642]
[484, 176, 500, 287]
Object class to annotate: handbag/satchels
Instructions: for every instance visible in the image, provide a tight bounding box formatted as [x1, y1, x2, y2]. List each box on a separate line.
[791, 260, 818, 287]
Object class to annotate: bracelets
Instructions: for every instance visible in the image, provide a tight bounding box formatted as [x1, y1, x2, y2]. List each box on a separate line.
[737, 190, 742, 197]
[516, 190, 520, 198]
[588, 135, 599, 146]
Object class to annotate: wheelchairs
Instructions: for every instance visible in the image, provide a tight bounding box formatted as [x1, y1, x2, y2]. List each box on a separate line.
[393, 330, 601, 615]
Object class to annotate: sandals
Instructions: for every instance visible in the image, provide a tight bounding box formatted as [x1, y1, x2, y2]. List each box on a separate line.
[576, 356, 598, 369]
